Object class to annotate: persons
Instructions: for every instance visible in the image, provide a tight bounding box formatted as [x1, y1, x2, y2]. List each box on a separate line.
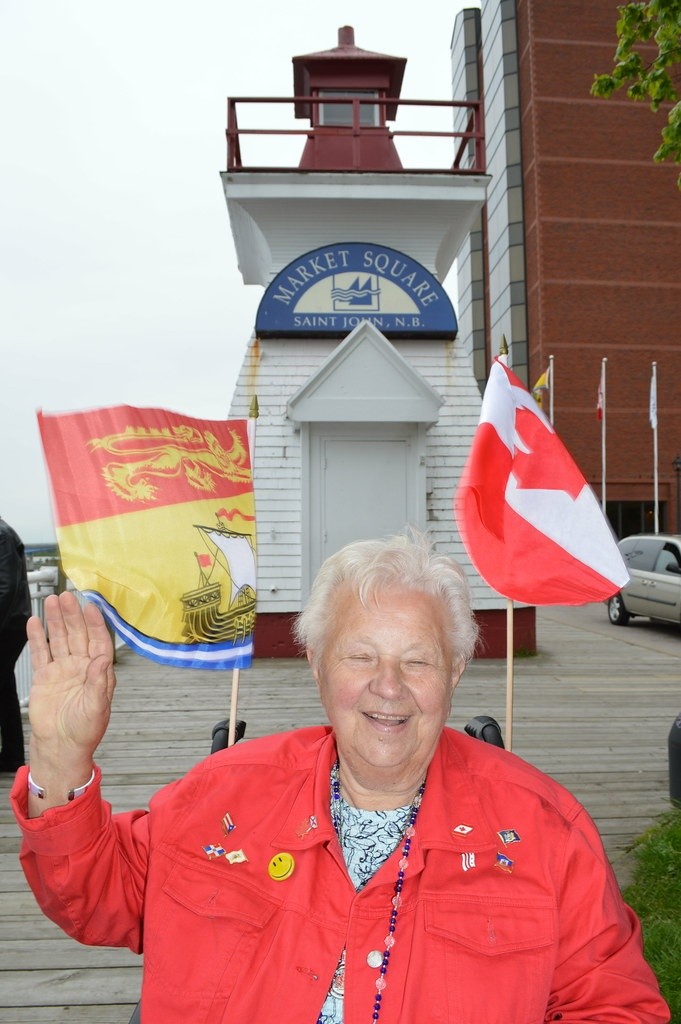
[0, 519, 32, 773]
[8, 538, 671, 1024]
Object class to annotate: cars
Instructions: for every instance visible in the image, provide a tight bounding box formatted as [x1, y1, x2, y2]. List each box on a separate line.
[602, 531, 681, 628]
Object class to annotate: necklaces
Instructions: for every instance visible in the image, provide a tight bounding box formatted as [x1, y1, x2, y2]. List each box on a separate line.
[333, 761, 425, 1024]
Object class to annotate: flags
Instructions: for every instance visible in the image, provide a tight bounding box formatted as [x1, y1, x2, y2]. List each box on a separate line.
[649, 377, 656, 427]
[37, 404, 257, 669]
[531, 367, 549, 408]
[456, 357, 630, 607]
[596, 378, 603, 421]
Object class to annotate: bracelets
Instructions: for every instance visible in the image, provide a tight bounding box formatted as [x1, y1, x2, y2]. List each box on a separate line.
[27, 768, 95, 803]
